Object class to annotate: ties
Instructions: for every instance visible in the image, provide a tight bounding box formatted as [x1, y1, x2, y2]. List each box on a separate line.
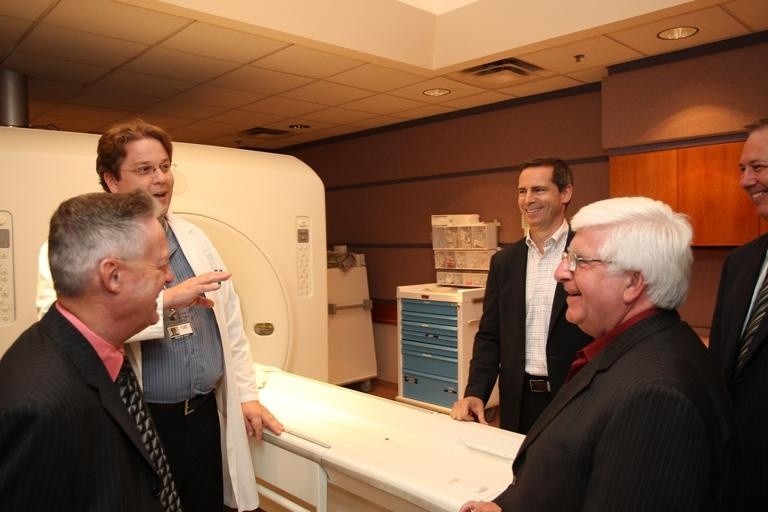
[116, 355, 185, 512]
[731, 267, 768, 382]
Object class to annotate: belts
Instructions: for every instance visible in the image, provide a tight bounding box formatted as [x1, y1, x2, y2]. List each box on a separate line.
[147, 387, 217, 417]
[523, 374, 551, 394]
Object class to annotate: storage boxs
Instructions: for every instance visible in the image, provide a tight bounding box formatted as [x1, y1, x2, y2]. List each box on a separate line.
[431, 222, 498, 288]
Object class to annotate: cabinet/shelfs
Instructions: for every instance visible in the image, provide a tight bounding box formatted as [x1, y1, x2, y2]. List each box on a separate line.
[397, 282, 500, 417]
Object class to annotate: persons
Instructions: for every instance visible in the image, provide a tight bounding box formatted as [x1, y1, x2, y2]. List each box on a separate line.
[707, 115, 768, 512]
[35, 118, 284, 512]
[450, 158, 586, 435]
[459, 196, 731, 512]
[0, 188, 175, 512]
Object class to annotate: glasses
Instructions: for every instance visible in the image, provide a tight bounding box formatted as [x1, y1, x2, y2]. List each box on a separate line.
[563, 251, 613, 271]
[118, 162, 178, 177]
